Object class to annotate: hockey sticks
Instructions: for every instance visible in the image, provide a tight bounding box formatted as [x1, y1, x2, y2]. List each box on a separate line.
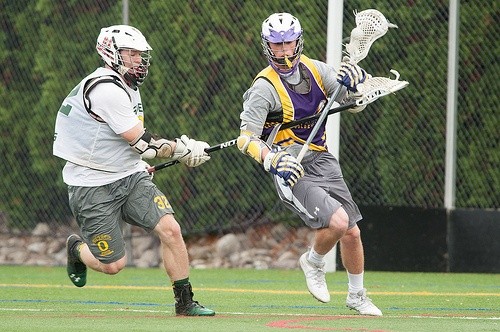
[139, 74, 410, 172]
[282, 9, 390, 188]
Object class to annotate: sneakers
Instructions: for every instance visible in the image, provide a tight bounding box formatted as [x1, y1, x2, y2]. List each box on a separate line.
[346, 288, 382, 316]
[66, 234, 86, 288]
[173, 285, 216, 316]
[299, 251, 330, 303]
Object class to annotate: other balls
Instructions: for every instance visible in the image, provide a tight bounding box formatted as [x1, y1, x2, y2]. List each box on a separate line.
[351, 27, 365, 40]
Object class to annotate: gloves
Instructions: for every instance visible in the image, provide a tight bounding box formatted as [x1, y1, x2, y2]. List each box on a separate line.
[142, 161, 154, 180]
[263, 150, 305, 187]
[170, 135, 211, 167]
[336, 56, 368, 93]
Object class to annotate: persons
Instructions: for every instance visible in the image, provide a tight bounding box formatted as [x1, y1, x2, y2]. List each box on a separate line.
[237, 14, 384, 315]
[53, 25, 216, 317]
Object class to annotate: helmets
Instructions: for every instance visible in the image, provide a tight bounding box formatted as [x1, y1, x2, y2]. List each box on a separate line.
[96, 25, 153, 73]
[262, 12, 304, 56]
